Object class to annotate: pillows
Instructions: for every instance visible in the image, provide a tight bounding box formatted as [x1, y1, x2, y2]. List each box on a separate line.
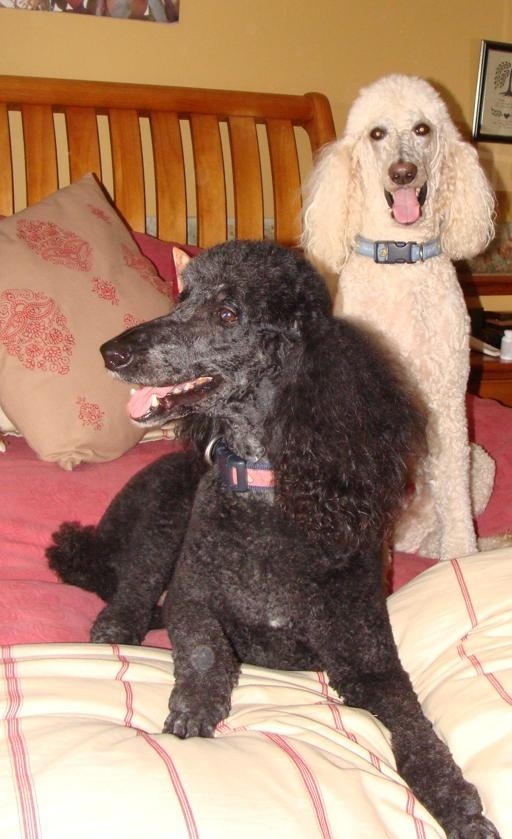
[0, 174, 212, 470]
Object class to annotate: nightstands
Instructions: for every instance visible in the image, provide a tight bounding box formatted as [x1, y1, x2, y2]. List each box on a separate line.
[467, 348, 512, 409]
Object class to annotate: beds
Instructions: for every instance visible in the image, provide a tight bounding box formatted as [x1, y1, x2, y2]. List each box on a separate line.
[0, 74, 512, 839]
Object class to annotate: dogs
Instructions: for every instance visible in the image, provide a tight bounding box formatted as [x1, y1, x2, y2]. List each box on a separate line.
[296, 69, 500, 557]
[42, 241, 496, 839]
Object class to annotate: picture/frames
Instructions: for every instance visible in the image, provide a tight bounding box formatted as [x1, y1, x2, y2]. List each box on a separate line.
[471, 37, 512, 145]
[451, 189, 512, 296]
